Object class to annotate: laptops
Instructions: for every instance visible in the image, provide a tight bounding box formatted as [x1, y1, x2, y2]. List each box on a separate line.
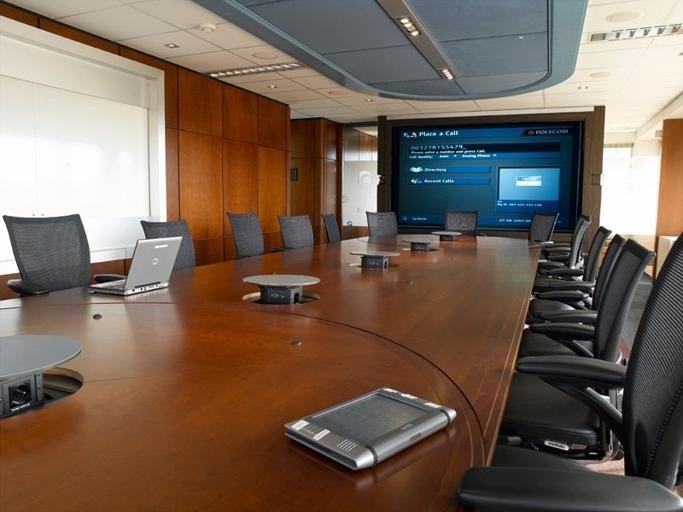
[87, 235, 184, 298]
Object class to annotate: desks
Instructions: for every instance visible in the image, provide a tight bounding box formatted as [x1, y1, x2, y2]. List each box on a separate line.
[0, 234, 542, 510]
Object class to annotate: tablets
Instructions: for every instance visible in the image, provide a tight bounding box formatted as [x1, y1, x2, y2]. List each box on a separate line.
[283, 384, 458, 472]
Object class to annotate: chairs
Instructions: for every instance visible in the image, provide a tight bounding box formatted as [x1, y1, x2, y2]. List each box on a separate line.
[321, 212, 340, 245]
[142, 218, 197, 272]
[446, 211, 478, 237]
[279, 215, 313, 251]
[225, 211, 290, 261]
[4, 212, 127, 297]
[457, 210, 682, 511]
[366, 212, 399, 239]
